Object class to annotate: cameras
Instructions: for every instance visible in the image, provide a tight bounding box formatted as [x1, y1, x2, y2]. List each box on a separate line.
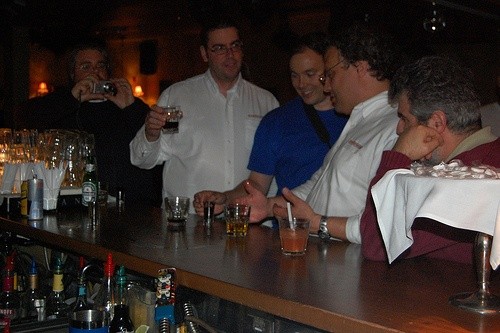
[92, 81, 114, 94]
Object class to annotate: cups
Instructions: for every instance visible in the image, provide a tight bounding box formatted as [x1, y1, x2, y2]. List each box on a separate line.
[278, 217, 311, 255]
[0, 127, 90, 187]
[116, 185, 127, 207]
[225, 201, 250, 237]
[162, 105, 180, 134]
[43, 190, 59, 210]
[203, 200, 215, 222]
[164, 196, 190, 226]
[97, 182, 109, 205]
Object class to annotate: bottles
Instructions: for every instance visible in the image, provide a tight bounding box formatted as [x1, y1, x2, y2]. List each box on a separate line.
[27, 174, 44, 220]
[0, 231, 175, 333]
[82, 147, 98, 207]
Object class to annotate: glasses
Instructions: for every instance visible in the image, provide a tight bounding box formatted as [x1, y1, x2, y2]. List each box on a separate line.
[319, 60, 347, 84]
[74, 62, 109, 73]
[208, 40, 244, 55]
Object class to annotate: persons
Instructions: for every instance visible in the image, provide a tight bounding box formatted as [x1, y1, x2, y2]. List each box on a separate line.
[129, 18, 281, 229]
[17, 38, 164, 208]
[193, 23, 500, 267]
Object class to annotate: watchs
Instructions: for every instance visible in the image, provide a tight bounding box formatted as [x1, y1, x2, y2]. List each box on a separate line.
[318, 216, 332, 241]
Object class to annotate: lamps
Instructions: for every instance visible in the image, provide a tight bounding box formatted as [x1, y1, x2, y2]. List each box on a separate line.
[35, 81, 49, 96]
[423, 0, 448, 34]
[132, 83, 145, 98]
